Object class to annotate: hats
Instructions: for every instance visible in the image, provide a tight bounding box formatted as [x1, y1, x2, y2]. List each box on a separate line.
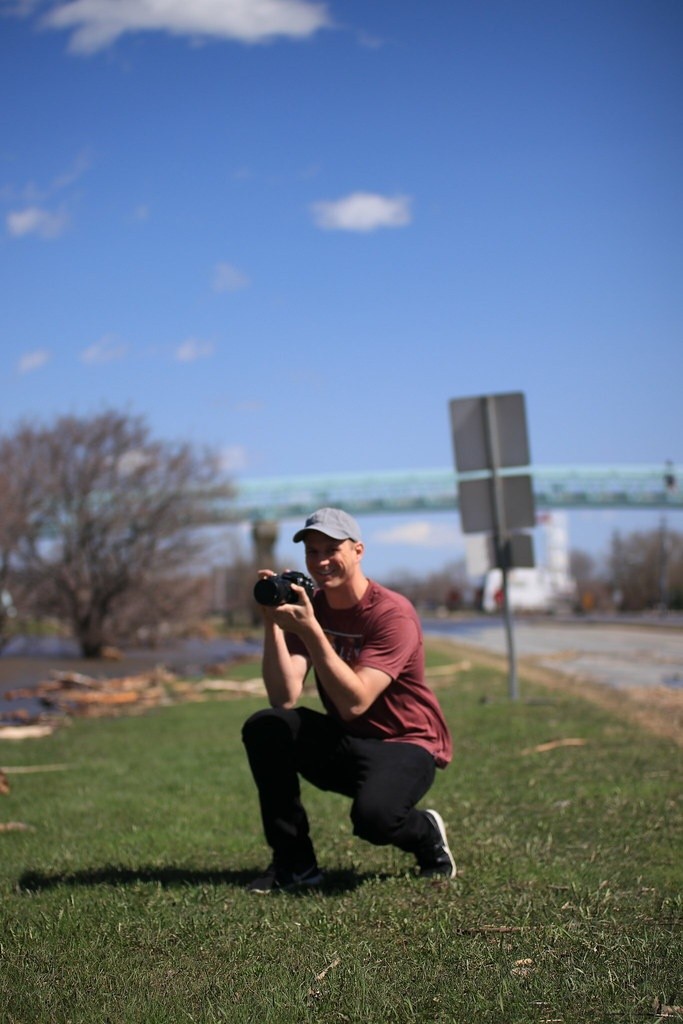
[293, 508, 362, 543]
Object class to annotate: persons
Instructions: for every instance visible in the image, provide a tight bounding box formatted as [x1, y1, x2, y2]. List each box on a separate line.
[241, 507, 461, 895]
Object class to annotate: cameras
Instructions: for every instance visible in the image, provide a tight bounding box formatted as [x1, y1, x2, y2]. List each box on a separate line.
[254, 571, 316, 608]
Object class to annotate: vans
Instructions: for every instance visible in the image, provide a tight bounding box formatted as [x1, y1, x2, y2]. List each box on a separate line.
[483, 571, 575, 613]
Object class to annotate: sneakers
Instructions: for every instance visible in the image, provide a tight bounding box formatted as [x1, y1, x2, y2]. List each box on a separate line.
[249, 863, 324, 894]
[418, 810, 456, 881]
